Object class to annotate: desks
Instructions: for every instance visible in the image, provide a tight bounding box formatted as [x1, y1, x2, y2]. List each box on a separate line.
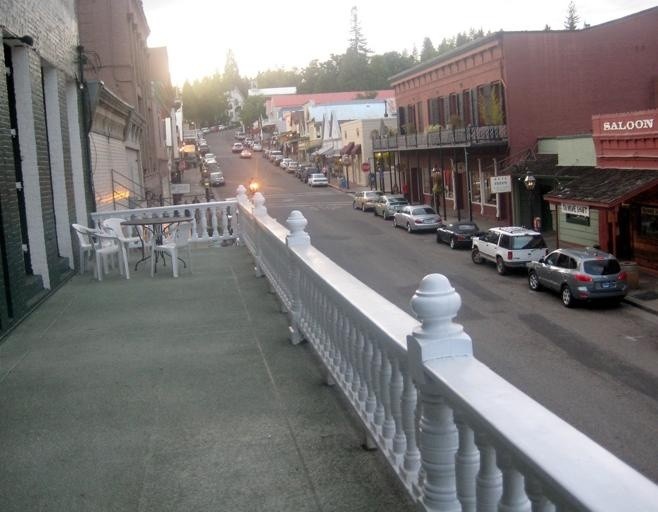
[119, 218, 193, 273]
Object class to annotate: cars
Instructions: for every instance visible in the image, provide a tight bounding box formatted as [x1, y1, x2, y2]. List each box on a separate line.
[393, 203, 442, 233]
[374, 193, 409, 220]
[352, 191, 382, 212]
[437, 222, 480, 249]
[264, 150, 329, 187]
[197, 124, 228, 187]
[231, 129, 262, 158]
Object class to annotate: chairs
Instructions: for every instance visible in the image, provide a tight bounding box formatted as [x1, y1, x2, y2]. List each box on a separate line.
[71, 217, 190, 282]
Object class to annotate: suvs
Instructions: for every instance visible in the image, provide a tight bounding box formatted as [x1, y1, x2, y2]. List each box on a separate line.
[527, 248, 628, 307]
[471, 226, 549, 275]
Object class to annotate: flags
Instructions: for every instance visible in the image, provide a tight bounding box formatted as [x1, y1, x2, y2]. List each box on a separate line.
[489, 174, 512, 194]
